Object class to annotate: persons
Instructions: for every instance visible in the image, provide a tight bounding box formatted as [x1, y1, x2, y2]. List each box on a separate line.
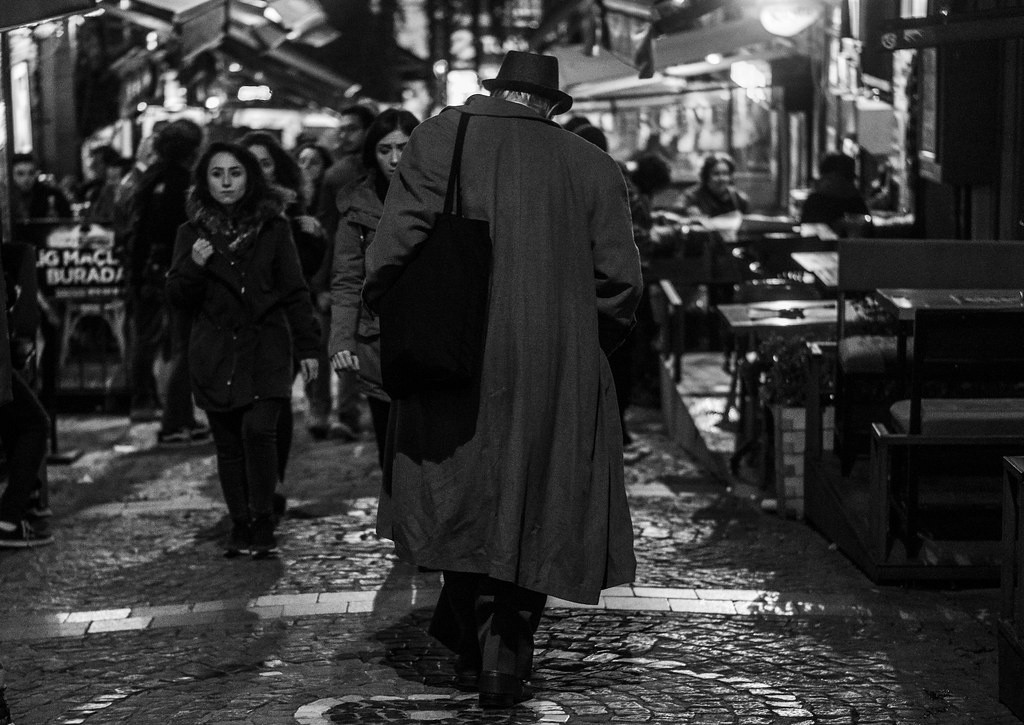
[0, 106, 421, 557]
[563, 117, 676, 457]
[800, 151, 872, 231]
[673, 157, 751, 268]
[361, 50, 646, 707]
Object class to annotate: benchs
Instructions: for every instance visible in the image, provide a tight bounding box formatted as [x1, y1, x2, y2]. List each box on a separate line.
[891, 305, 1024, 557]
[834, 239, 1024, 478]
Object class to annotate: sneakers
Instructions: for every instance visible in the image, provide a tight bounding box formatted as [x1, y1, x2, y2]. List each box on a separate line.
[0, 519, 55, 547]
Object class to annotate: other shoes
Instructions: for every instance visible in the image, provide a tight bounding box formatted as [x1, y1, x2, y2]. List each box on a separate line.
[159, 422, 213, 448]
[223, 539, 268, 561]
[309, 420, 330, 440]
[331, 422, 357, 441]
[130, 406, 164, 422]
[453, 652, 483, 692]
[478, 671, 535, 708]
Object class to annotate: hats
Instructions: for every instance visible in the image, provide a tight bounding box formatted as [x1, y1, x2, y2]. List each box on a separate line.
[482, 50, 573, 115]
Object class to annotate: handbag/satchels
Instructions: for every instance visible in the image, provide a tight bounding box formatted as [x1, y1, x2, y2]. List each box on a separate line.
[378, 114, 493, 396]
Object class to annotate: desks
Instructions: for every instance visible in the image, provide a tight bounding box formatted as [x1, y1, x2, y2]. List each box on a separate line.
[716, 299, 887, 469]
[875, 288, 1024, 560]
